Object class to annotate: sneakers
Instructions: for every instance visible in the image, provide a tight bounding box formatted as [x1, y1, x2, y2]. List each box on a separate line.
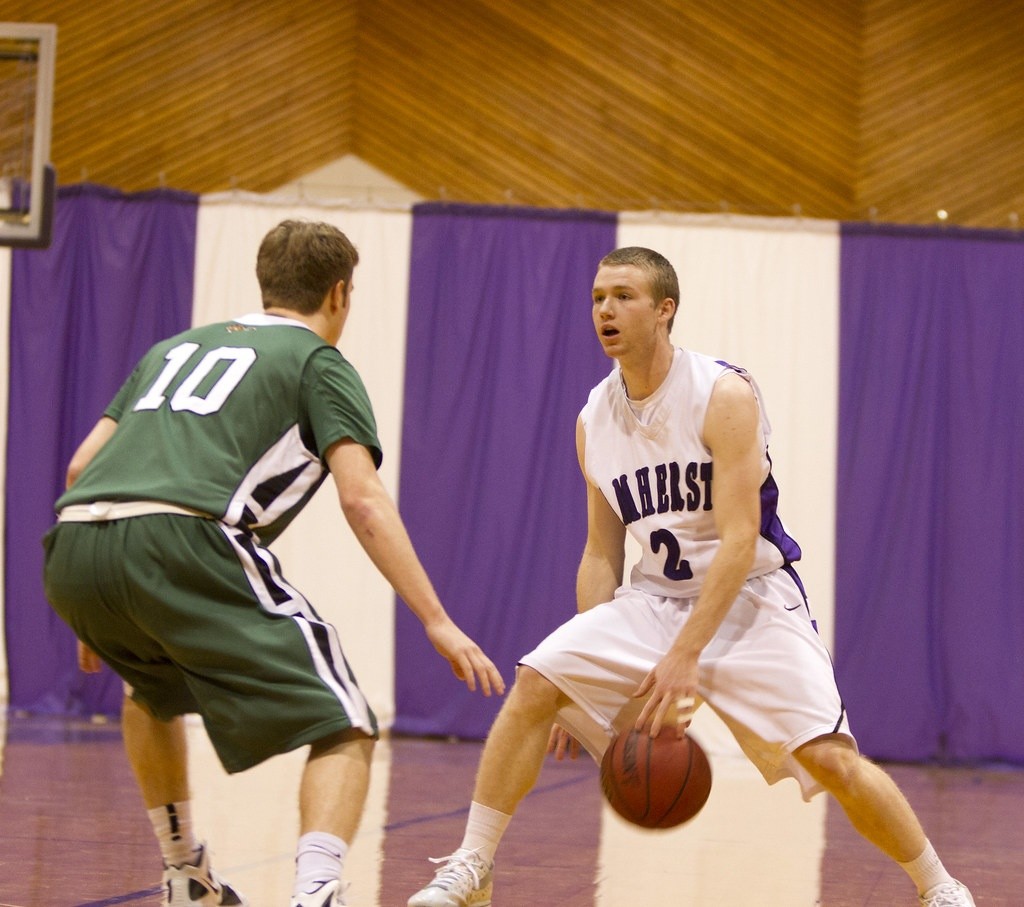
[407, 844, 494, 907]
[160, 844, 249, 907]
[919, 878, 976, 907]
[292, 879, 347, 907]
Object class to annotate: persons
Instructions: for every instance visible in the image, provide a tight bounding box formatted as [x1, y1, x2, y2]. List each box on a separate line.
[40, 217, 507, 907]
[406, 245, 977, 907]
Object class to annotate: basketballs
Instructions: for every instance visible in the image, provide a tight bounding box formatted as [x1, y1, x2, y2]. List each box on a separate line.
[597, 722, 715, 833]
